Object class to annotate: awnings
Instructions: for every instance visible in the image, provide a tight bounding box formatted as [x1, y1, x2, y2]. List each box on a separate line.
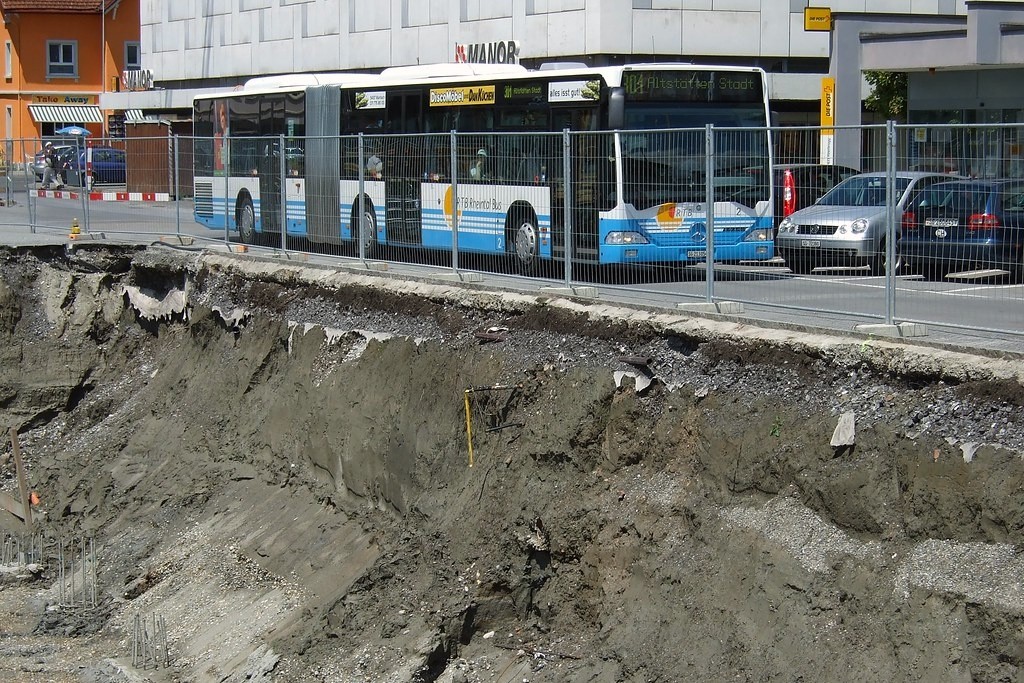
[28, 104, 104, 123]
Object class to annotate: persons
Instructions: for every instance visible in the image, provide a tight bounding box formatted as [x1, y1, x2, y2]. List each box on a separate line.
[197, 135, 755, 184]
[37, 142, 65, 191]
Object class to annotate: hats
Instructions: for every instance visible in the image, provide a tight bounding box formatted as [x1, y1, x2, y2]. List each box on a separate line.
[476, 150, 488, 157]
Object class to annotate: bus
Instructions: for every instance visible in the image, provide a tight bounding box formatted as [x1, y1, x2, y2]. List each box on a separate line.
[190, 60, 777, 278]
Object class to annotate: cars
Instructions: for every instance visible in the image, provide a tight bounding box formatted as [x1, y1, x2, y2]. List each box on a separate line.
[33, 145, 126, 188]
[736, 163, 861, 239]
[776, 173, 971, 275]
[896, 177, 1023, 283]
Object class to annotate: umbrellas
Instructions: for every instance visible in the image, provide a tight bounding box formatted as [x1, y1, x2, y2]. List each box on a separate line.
[56, 126, 92, 137]
